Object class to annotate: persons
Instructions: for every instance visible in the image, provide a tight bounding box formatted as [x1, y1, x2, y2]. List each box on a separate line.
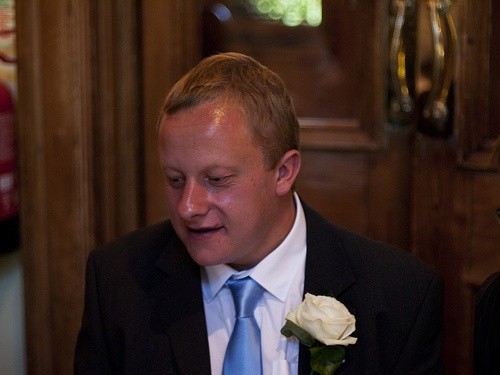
[71, 51, 457, 375]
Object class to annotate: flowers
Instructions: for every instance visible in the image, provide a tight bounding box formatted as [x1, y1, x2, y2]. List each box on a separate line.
[278, 293, 358, 374]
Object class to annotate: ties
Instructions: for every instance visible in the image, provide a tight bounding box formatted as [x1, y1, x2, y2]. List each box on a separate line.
[222, 277, 266, 375]
